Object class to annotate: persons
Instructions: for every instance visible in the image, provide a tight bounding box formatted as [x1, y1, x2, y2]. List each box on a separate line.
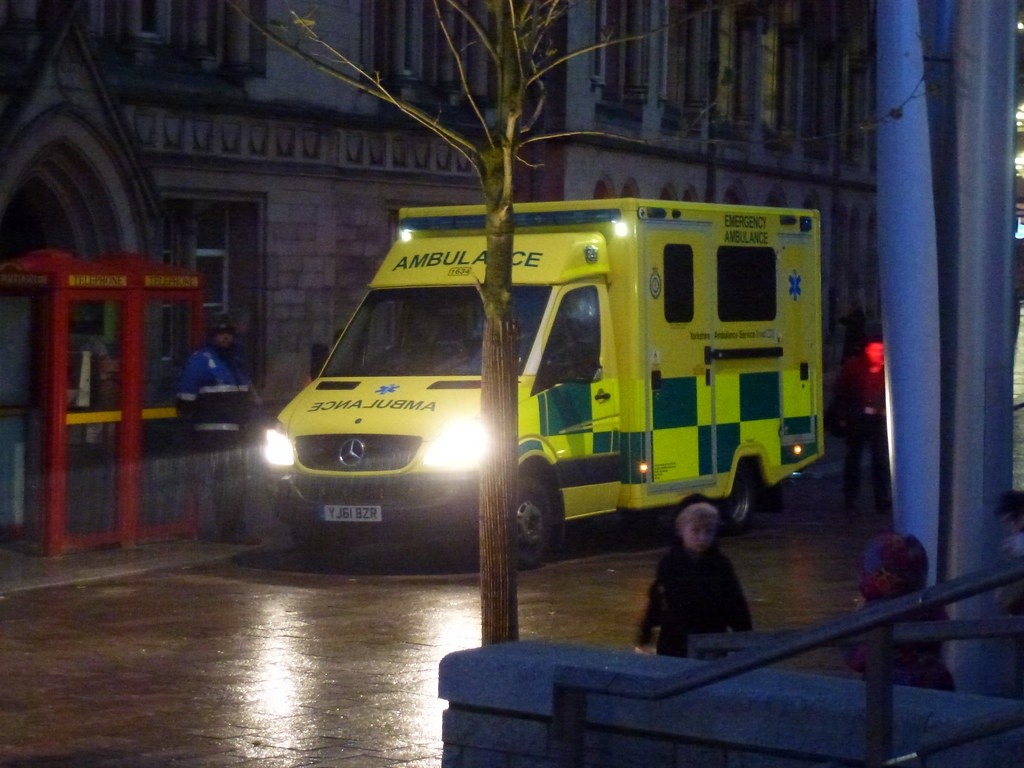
[997, 491, 1024, 613]
[826, 338, 890, 514]
[301, 306, 485, 385]
[70, 303, 262, 549]
[634, 491, 752, 658]
[847, 527, 960, 691]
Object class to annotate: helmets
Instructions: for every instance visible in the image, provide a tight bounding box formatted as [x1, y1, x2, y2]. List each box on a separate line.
[854, 532, 928, 603]
[202, 311, 236, 333]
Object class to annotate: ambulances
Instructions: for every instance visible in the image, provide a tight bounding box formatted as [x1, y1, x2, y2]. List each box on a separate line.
[259, 196, 826, 568]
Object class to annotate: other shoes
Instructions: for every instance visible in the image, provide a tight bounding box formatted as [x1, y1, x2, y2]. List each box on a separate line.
[222, 527, 256, 545]
[197, 529, 233, 543]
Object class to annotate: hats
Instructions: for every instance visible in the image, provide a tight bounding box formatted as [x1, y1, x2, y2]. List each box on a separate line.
[838, 313, 868, 332]
[670, 493, 723, 530]
